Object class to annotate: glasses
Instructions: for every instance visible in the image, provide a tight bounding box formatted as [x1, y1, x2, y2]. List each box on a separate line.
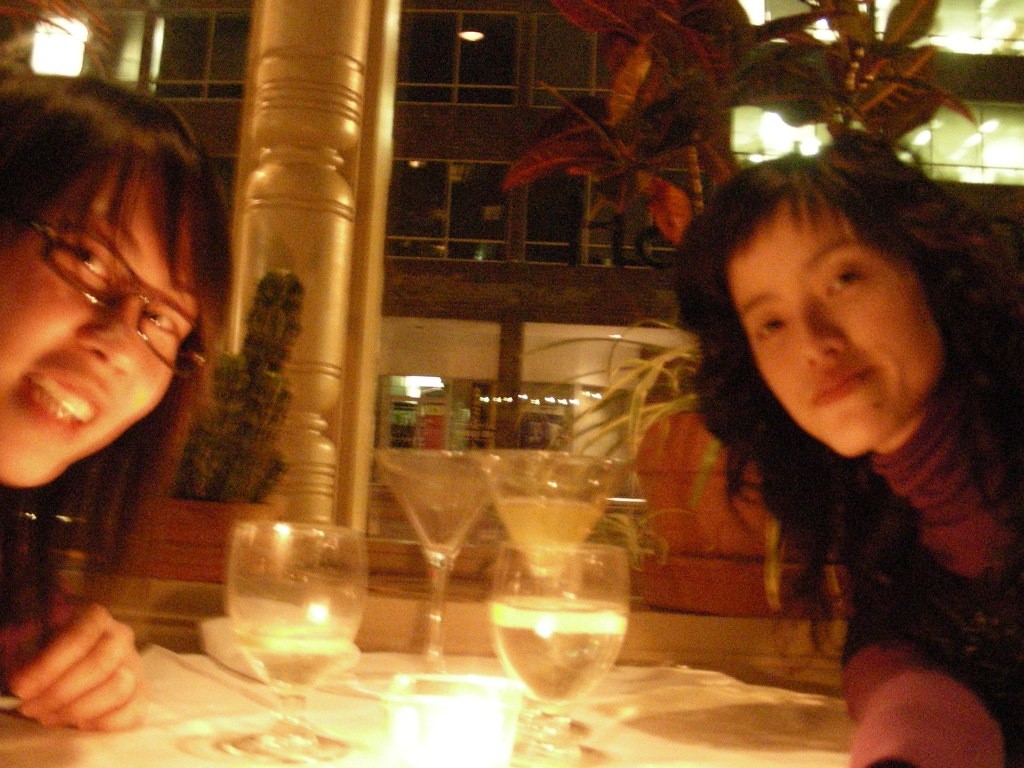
[12, 210, 213, 380]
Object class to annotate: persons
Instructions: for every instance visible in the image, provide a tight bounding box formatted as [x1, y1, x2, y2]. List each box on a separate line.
[668, 132, 1024, 767]
[0, 69, 231, 734]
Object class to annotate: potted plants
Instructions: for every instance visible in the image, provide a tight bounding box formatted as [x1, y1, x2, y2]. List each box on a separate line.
[128, 268, 304, 580]
[536, 325, 846, 614]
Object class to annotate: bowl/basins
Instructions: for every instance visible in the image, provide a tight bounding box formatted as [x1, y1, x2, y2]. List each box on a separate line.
[203, 617, 361, 682]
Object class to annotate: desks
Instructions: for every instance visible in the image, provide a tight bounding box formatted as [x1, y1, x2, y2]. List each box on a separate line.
[0, 662, 854, 768]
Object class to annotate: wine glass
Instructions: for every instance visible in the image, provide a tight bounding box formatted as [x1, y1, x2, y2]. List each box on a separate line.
[368, 448, 502, 698]
[490, 543, 630, 768]
[487, 449, 628, 740]
[226, 525, 369, 763]
